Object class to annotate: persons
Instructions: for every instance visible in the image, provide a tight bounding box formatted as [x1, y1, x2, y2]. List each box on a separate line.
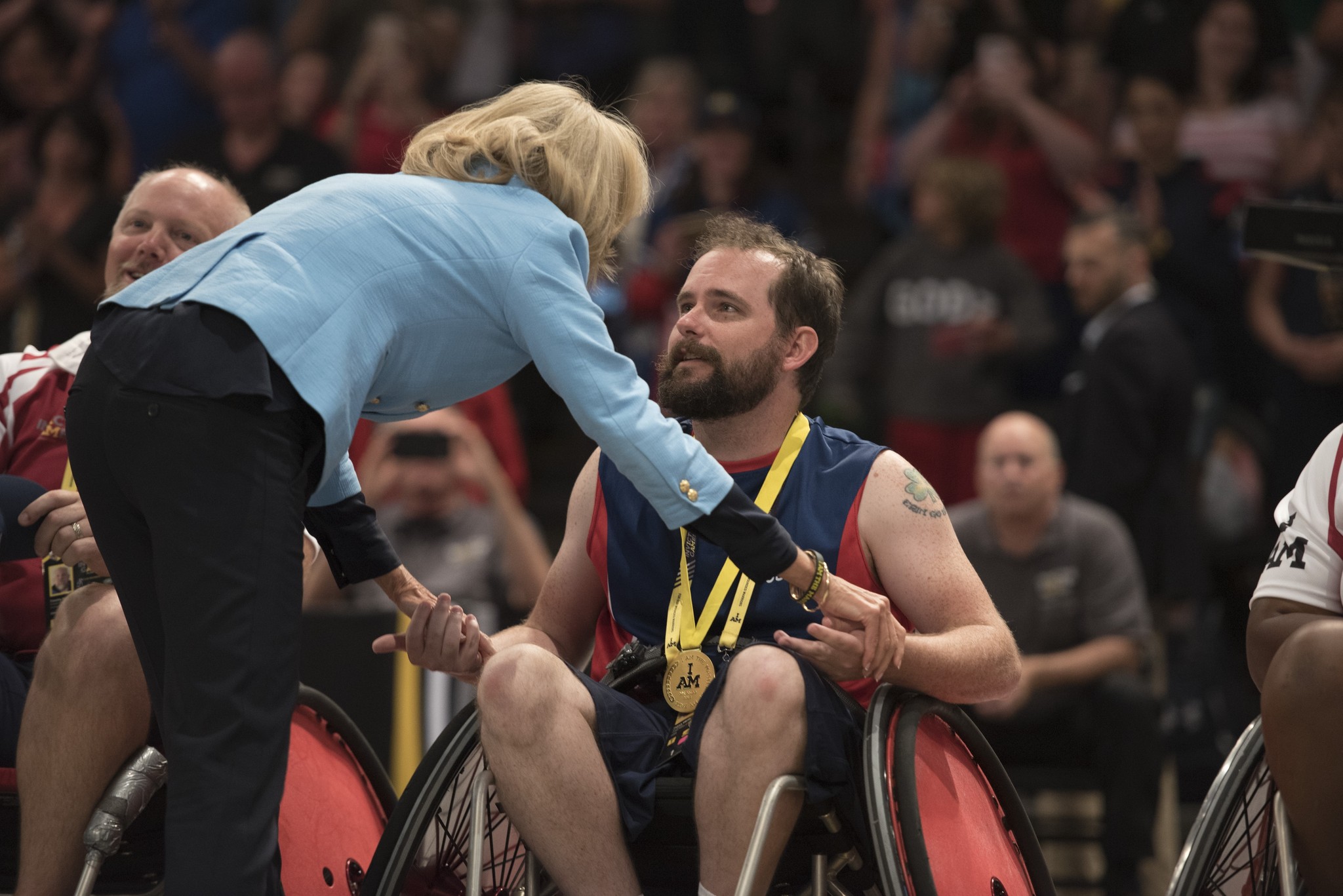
[0, 0, 1343, 896]
[946, 409, 1166, 896]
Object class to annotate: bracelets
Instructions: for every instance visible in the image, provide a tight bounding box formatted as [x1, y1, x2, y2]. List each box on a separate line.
[790, 548, 824, 606]
[802, 562, 830, 612]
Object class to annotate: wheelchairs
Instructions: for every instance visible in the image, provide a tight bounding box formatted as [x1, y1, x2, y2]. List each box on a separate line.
[72, 681, 416, 896]
[1170, 712, 1299, 895]
[355, 644, 1053, 896]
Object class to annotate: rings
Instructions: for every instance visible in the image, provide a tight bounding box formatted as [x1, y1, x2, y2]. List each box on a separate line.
[72, 522, 83, 539]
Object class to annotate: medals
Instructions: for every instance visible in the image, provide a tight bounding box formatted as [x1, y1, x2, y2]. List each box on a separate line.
[661, 651, 716, 714]
